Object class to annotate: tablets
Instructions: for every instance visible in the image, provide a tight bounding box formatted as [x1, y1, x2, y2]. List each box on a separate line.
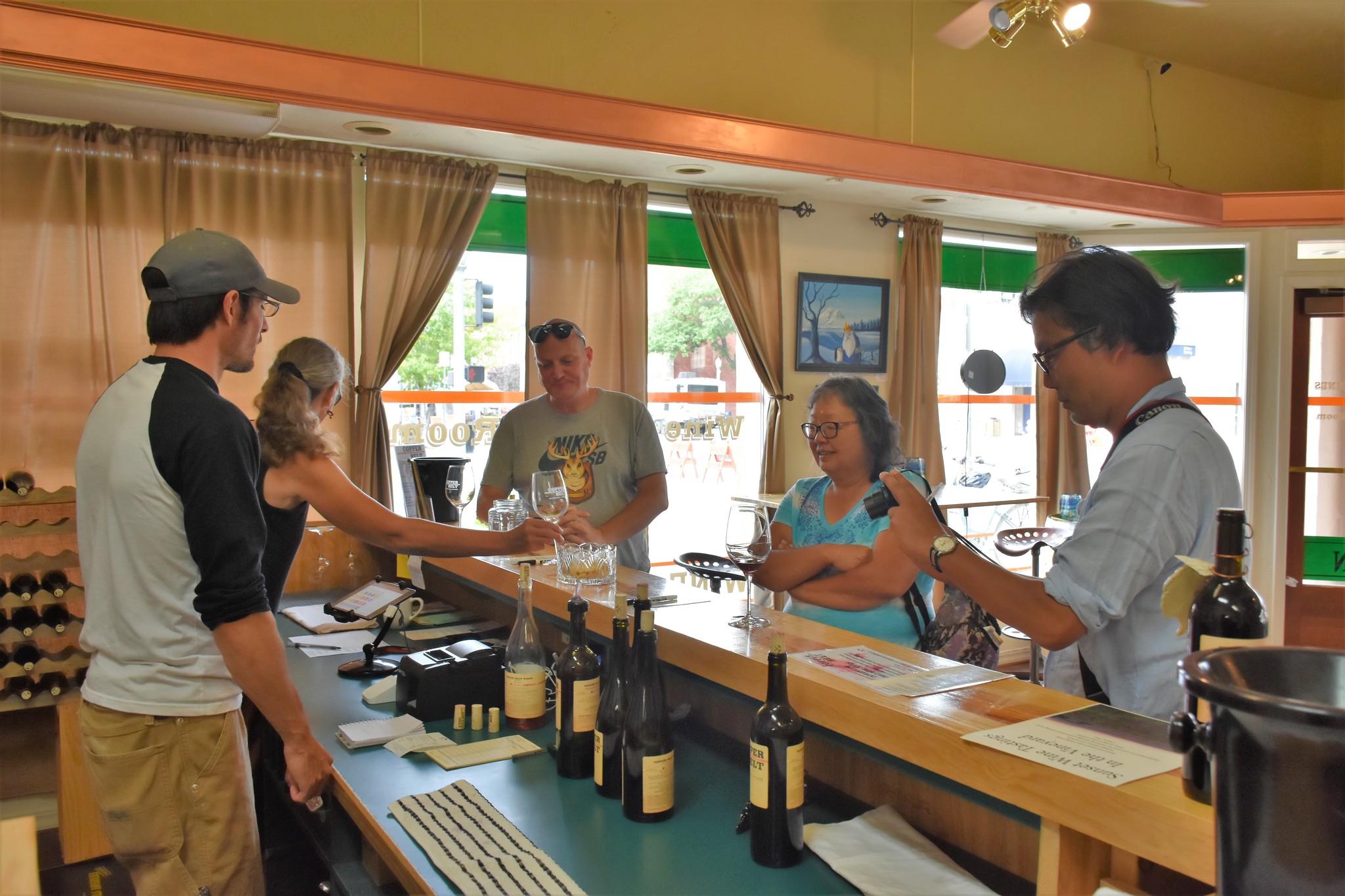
[331, 580, 416, 620]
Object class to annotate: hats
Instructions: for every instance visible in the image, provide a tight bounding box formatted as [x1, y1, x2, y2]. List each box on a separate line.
[141, 228, 301, 304]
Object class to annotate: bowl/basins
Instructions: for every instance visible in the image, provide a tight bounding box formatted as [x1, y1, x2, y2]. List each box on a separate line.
[553, 538, 617, 585]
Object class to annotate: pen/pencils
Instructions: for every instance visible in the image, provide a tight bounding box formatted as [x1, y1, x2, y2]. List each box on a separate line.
[287, 642, 342, 649]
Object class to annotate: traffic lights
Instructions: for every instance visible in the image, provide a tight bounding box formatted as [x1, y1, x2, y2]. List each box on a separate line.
[476, 281, 495, 327]
[465, 366, 485, 383]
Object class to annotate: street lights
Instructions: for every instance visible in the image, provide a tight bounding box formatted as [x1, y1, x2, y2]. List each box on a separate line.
[715, 356, 722, 379]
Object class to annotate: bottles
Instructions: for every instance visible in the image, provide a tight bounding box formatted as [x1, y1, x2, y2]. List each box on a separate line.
[556, 582, 599, 780]
[488, 498, 528, 532]
[748, 628, 805, 869]
[1182, 506, 1269, 805]
[621, 609, 675, 823]
[5, 467, 36, 495]
[504, 563, 546, 731]
[630, 583, 652, 653]
[0, 566, 68, 701]
[594, 594, 634, 800]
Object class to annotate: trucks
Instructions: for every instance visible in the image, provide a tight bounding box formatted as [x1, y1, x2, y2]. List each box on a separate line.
[647, 352, 726, 434]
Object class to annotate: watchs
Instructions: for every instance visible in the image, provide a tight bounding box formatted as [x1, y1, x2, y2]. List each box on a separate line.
[928, 533, 961, 574]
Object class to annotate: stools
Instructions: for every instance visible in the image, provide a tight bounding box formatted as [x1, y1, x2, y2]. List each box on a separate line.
[994, 526, 1067, 685]
[673, 552, 766, 595]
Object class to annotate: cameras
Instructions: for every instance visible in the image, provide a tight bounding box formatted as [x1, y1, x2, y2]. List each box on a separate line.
[863, 482, 899, 521]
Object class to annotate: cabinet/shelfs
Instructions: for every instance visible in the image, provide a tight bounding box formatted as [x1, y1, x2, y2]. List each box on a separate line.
[0, 487, 92, 711]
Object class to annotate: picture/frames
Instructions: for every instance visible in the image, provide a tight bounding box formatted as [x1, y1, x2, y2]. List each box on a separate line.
[794, 271, 892, 374]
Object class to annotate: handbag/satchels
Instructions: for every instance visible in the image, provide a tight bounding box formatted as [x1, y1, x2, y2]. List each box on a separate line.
[901, 471, 1001, 670]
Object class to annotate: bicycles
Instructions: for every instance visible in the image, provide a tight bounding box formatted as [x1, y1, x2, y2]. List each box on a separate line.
[944, 448, 1036, 547]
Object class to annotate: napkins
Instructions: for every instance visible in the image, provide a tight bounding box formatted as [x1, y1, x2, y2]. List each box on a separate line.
[282, 603, 379, 633]
[803, 805, 1003, 896]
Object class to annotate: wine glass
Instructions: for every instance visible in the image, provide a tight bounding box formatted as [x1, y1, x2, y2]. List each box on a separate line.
[336, 533, 366, 595]
[531, 470, 569, 565]
[725, 504, 771, 630]
[305, 527, 335, 598]
[446, 464, 475, 528]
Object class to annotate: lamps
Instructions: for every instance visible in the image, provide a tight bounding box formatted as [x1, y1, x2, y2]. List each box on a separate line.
[988, 0, 1090, 48]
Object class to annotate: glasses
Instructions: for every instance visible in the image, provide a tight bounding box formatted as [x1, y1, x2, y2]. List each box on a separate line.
[801, 421, 859, 439]
[1032, 324, 1099, 375]
[238, 291, 279, 318]
[528, 322, 586, 347]
[334, 395, 341, 406]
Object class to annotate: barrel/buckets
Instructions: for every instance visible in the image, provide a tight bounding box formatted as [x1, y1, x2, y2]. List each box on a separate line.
[409, 456, 471, 522]
[1166, 646, 1344, 896]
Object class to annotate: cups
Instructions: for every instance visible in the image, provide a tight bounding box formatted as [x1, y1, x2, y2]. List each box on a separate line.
[1060, 494, 1083, 521]
[904, 457, 925, 479]
[383, 597, 424, 630]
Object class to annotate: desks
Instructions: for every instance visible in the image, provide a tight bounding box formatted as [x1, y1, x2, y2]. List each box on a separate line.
[731, 487, 1051, 612]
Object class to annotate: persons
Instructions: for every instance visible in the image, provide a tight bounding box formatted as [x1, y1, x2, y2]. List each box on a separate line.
[878, 244, 1252, 727]
[244, 335, 566, 896]
[77, 229, 336, 895]
[477, 320, 670, 571]
[746, 378, 942, 653]
